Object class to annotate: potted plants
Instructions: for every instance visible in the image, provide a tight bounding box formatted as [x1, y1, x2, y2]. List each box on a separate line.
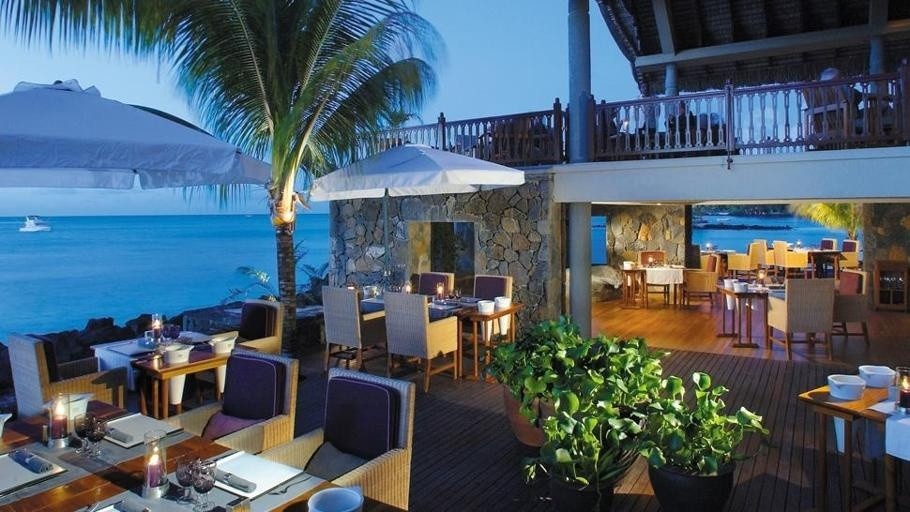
[528, 357, 666, 512]
[637, 370, 770, 511]
[487, 314, 598, 449]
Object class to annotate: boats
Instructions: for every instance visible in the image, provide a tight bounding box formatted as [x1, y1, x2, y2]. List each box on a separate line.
[16, 210, 49, 236]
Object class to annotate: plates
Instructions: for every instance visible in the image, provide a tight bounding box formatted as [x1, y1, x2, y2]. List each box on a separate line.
[42, 392, 94, 421]
[0, 413, 12, 439]
[210, 331, 238, 353]
[165, 344, 195, 365]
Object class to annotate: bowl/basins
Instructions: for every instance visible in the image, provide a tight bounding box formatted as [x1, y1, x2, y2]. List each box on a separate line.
[495, 297, 511, 308]
[478, 300, 495, 313]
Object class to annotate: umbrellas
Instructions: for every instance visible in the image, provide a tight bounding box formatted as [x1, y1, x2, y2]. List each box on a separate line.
[305, 143, 528, 292]
[1, 78, 275, 193]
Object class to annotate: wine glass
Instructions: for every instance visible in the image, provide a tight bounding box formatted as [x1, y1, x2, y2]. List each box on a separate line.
[171, 323, 181, 345]
[455, 289, 462, 299]
[162, 323, 170, 341]
[188, 459, 217, 512]
[176, 455, 196, 505]
[74, 412, 92, 457]
[89, 420, 107, 456]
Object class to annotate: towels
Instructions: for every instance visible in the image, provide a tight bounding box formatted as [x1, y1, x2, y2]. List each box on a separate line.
[137, 338, 154, 350]
[435, 301, 457, 306]
[9, 445, 55, 474]
[215, 469, 256, 495]
[105, 428, 132, 444]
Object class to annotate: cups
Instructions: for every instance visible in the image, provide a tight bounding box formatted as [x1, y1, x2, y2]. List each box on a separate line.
[307, 485, 363, 512]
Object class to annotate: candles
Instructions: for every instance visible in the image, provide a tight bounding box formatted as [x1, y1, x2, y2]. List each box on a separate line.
[147, 447, 162, 488]
[437, 286, 444, 299]
[900, 379, 910, 408]
[51, 402, 68, 439]
[151, 320, 161, 339]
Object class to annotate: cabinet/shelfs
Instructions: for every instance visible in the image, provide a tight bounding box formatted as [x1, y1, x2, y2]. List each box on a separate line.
[872, 260, 910, 313]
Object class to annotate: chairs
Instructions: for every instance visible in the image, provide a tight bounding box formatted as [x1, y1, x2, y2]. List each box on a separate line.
[384, 295, 459, 393]
[727, 243, 760, 284]
[322, 285, 386, 372]
[753, 239, 776, 279]
[798, 79, 899, 150]
[821, 240, 837, 274]
[201, 298, 284, 398]
[838, 239, 859, 271]
[5, 331, 129, 421]
[637, 251, 666, 296]
[256, 367, 416, 510]
[160, 347, 300, 453]
[773, 240, 810, 281]
[420, 273, 455, 358]
[684, 254, 720, 305]
[473, 275, 514, 348]
[825, 269, 869, 346]
[765, 279, 836, 362]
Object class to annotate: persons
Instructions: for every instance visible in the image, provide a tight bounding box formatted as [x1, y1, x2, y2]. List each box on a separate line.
[631, 82, 662, 160]
[816, 66, 872, 136]
[595, 101, 624, 139]
[665, 100, 700, 159]
